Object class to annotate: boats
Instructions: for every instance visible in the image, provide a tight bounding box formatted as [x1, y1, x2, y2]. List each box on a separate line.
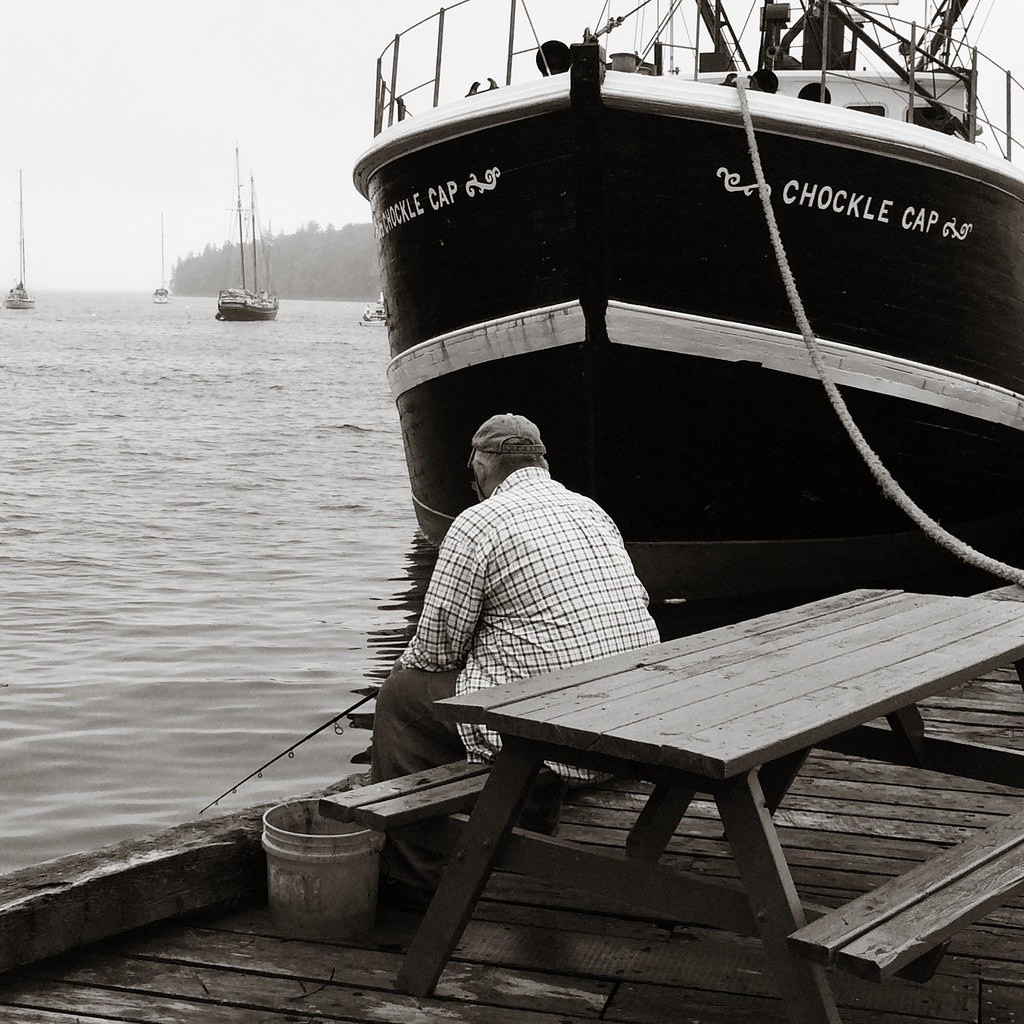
[358, 292, 386, 327]
[352, 0, 1024, 592]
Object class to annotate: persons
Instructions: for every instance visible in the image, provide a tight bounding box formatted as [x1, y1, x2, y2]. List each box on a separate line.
[371, 412, 661, 914]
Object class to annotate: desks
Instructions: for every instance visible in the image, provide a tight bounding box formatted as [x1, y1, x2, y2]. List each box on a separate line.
[392, 586, 1024, 1024]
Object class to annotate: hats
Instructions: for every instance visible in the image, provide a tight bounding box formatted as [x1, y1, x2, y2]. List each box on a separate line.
[467, 413, 546, 468]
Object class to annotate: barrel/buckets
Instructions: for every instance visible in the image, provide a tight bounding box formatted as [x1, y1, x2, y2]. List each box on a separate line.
[261, 798, 386, 940]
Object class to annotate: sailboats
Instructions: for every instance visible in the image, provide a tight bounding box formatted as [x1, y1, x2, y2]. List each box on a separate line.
[218, 147, 279, 320]
[154, 211, 169, 303]
[5, 168, 36, 309]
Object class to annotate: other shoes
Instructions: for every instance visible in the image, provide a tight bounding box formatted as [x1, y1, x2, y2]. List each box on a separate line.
[377, 872, 434, 913]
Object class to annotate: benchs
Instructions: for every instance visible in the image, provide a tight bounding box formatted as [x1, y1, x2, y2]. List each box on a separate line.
[785, 806, 1024, 984]
[317, 760, 491, 854]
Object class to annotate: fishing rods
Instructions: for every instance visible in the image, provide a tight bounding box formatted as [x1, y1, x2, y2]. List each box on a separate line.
[200, 685, 381, 812]
